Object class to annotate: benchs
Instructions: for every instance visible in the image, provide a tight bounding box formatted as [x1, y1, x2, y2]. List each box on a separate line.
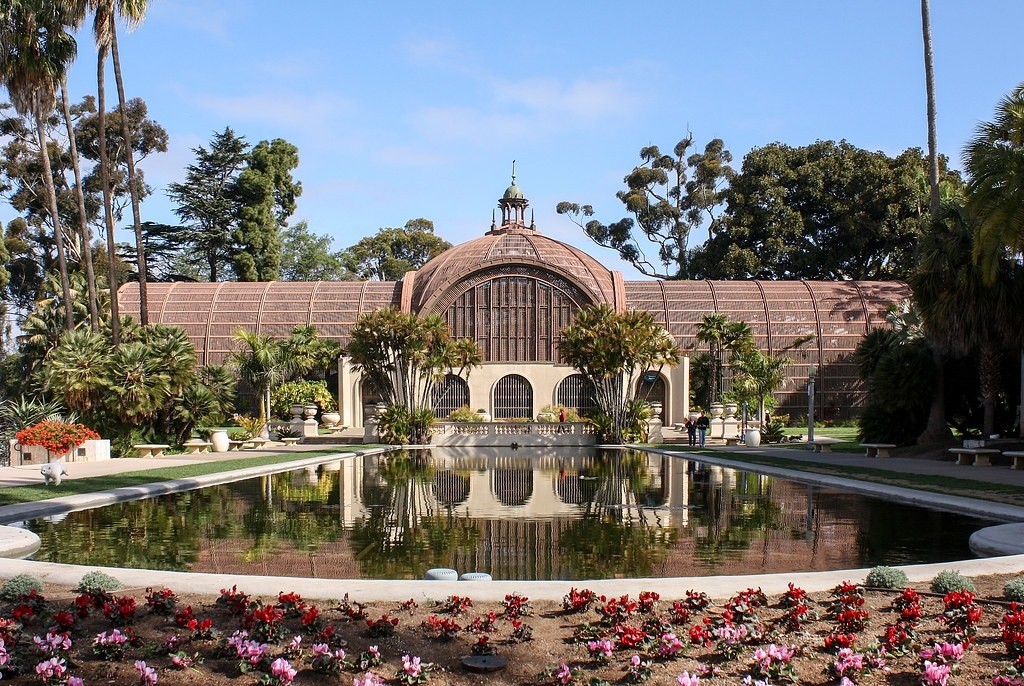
[183, 443, 214, 454]
[859, 444, 896, 458]
[1002, 452, 1024, 470]
[281, 438, 301, 446]
[723, 437, 740, 446]
[229, 440, 270, 452]
[329, 425, 350, 434]
[134, 445, 169, 458]
[808, 442, 837, 453]
[674, 423, 687, 431]
[948, 448, 1000, 466]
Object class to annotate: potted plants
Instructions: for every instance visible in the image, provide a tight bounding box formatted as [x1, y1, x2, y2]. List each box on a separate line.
[537, 406, 554, 422]
[474, 408, 491, 422]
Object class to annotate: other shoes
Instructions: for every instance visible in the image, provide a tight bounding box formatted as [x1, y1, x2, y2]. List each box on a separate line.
[693, 445, 695, 447]
[703, 445, 705, 448]
[689, 445, 692, 448]
[700, 445, 702, 448]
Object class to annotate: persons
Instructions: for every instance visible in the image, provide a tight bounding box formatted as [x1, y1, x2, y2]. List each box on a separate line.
[685, 411, 709, 448]
[556, 409, 567, 434]
